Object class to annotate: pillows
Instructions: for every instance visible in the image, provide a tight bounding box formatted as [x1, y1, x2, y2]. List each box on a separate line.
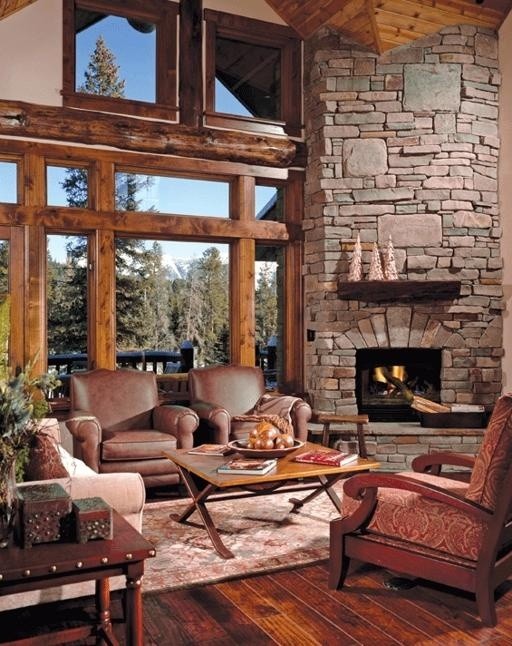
[26, 429, 70, 481]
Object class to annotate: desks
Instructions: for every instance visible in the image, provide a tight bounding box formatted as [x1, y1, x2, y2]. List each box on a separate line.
[0, 507, 156, 646]
[162, 438, 381, 559]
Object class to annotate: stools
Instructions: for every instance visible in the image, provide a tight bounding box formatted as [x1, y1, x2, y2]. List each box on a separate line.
[318, 413, 369, 459]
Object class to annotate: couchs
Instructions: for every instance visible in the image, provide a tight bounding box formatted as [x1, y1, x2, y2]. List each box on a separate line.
[0, 417, 146, 613]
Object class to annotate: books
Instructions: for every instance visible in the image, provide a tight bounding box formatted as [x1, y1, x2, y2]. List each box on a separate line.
[188, 443, 235, 458]
[294, 450, 359, 467]
[218, 456, 279, 475]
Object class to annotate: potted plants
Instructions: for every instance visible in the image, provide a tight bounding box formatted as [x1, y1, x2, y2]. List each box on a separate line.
[0, 347, 62, 549]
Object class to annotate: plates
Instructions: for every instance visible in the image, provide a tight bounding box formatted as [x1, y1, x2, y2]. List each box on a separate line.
[228, 438, 305, 459]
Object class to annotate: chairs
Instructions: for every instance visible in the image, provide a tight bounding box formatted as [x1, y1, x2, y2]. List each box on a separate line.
[188, 362, 312, 448]
[46, 367, 200, 498]
[329, 392, 512, 628]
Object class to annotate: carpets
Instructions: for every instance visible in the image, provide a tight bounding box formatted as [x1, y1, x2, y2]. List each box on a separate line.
[141, 478, 350, 594]
